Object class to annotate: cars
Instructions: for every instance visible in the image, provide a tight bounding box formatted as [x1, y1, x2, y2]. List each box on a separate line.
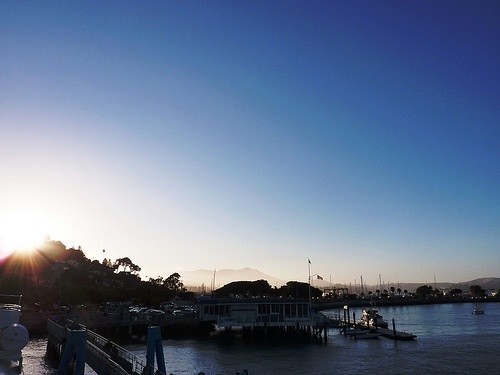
[34, 296, 201, 326]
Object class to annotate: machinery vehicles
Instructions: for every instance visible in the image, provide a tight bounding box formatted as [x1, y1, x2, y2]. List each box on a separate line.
[0, 304, 29, 373]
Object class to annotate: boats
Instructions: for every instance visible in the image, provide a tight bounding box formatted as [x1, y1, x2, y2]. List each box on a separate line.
[361, 308, 388, 328]
[472, 308, 484, 315]
